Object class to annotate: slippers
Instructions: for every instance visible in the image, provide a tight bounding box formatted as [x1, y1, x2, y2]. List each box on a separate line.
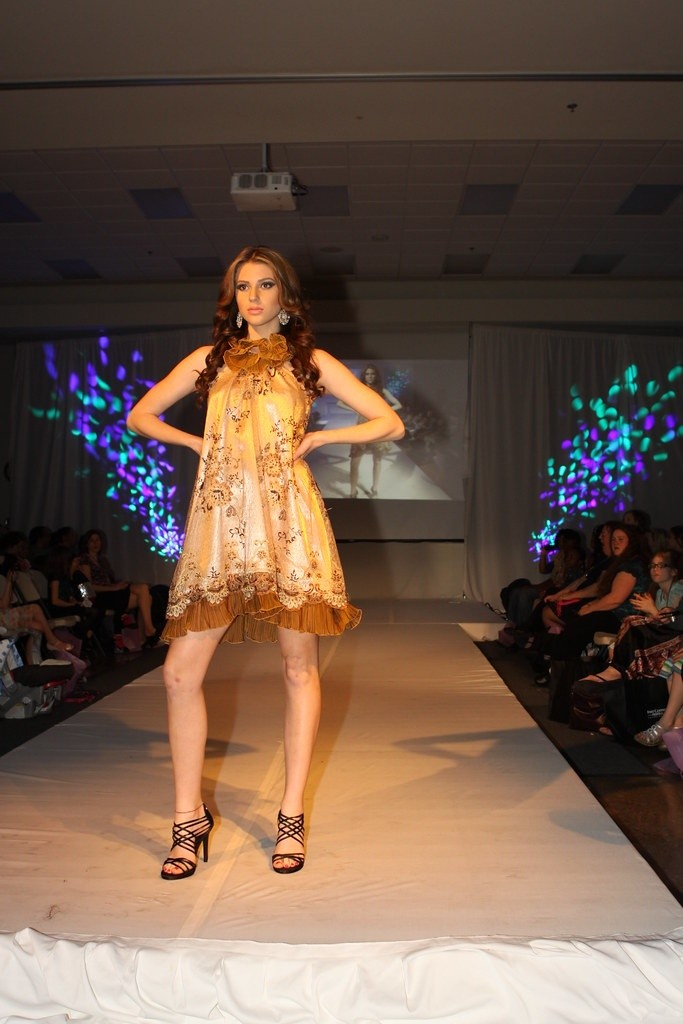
[634, 723, 683, 751]
[146, 630, 163, 643]
[577, 671, 624, 690]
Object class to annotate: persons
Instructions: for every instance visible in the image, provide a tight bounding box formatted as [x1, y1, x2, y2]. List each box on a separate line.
[397, 404, 447, 458]
[0, 525, 160, 720]
[337, 363, 404, 498]
[125, 245, 404, 880]
[499, 509, 683, 749]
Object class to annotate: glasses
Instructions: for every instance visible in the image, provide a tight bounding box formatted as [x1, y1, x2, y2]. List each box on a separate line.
[648, 562, 674, 570]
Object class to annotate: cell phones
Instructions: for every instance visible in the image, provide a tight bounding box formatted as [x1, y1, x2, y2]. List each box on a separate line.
[78, 583, 88, 597]
[544, 545, 559, 552]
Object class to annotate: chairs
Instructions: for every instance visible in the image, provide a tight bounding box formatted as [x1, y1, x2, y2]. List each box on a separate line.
[592, 630, 617, 645]
[0, 570, 82, 629]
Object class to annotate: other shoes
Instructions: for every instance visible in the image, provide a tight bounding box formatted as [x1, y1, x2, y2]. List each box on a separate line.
[522, 635, 537, 651]
[536, 672, 551, 685]
[47, 639, 74, 651]
[114, 646, 129, 655]
[599, 726, 614, 735]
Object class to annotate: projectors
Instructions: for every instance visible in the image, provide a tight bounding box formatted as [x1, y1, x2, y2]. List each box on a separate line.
[230, 171, 300, 211]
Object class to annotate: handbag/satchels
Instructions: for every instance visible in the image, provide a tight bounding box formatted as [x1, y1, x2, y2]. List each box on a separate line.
[569, 685, 608, 728]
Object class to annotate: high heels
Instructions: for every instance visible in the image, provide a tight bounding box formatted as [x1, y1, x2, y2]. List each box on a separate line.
[371, 485, 378, 499]
[161, 803, 214, 880]
[271, 812, 307, 876]
[349, 489, 358, 504]
